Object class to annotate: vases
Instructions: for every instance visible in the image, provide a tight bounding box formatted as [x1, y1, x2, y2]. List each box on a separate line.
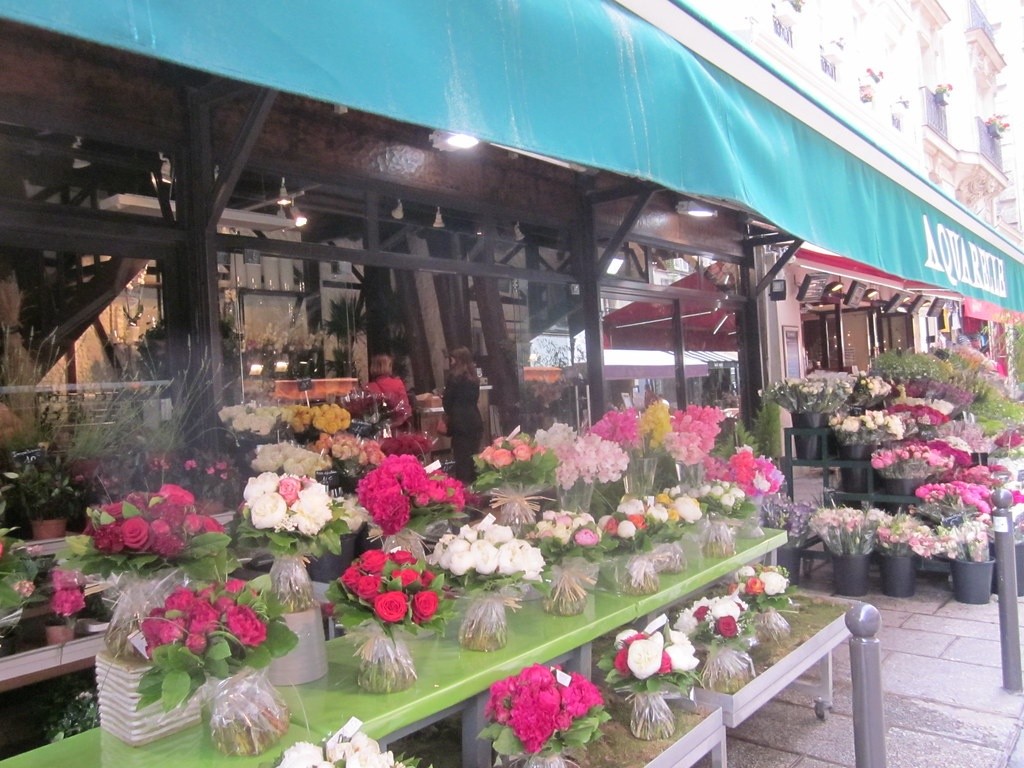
[838, 443, 880, 494]
[881, 555, 915, 598]
[29, 518, 67, 543]
[126, 326, 139, 344]
[46, 624, 73, 644]
[832, 555, 868, 596]
[948, 556, 996, 605]
[267, 605, 327, 686]
[776, 548, 801, 587]
[94, 650, 202, 749]
[790, 412, 831, 463]
[0, 631, 18, 658]
[885, 477, 922, 515]
[990, 540, 1023, 597]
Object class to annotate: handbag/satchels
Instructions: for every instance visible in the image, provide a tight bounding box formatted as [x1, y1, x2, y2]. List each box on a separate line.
[437, 413, 451, 434]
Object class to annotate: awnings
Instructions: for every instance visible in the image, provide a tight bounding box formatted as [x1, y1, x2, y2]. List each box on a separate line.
[0, 0, 1023, 317]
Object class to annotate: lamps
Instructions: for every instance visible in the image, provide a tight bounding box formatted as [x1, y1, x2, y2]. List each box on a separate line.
[824, 281, 844, 292]
[288, 198, 307, 227]
[769, 279, 786, 301]
[158, 152, 171, 184]
[513, 222, 524, 241]
[862, 289, 878, 298]
[432, 206, 445, 227]
[896, 304, 908, 313]
[70, 137, 91, 169]
[276, 176, 291, 205]
[674, 199, 717, 217]
[390, 198, 403, 219]
[428, 130, 479, 153]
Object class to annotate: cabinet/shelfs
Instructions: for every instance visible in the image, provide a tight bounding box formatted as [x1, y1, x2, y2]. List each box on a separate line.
[0, 511, 864, 768]
[778, 427, 922, 574]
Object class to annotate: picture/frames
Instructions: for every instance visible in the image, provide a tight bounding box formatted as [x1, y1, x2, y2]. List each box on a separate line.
[781, 326, 801, 379]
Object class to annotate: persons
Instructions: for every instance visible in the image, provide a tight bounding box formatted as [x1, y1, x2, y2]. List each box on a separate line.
[442, 344, 484, 488]
[363, 352, 412, 434]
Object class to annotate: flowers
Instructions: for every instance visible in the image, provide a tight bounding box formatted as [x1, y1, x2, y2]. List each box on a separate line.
[985, 113, 1009, 132]
[119, 263, 147, 326]
[0, 344, 1024, 768]
[934, 83, 953, 96]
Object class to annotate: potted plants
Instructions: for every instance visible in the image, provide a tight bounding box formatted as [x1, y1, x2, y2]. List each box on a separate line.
[935, 93, 949, 106]
[988, 125, 1004, 140]
